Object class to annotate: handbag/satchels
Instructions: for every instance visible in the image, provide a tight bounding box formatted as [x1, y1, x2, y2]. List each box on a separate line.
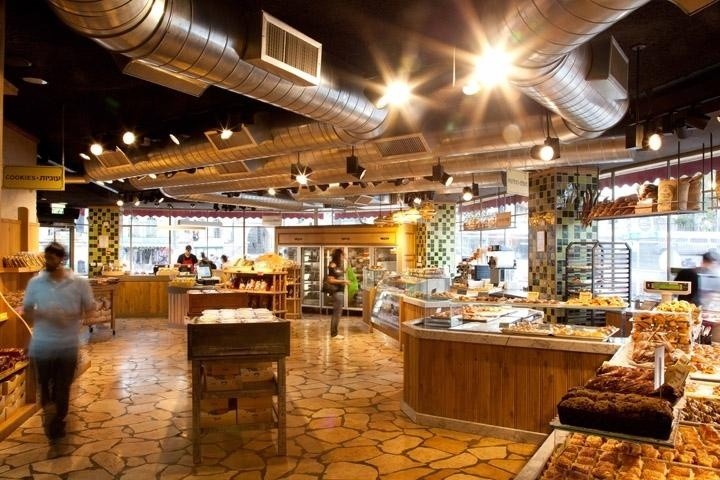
[321, 277, 345, 293]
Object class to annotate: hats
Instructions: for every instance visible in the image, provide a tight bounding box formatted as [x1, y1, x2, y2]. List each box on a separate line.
[186, 245, 192, 250]
[45, 241, 66, 257]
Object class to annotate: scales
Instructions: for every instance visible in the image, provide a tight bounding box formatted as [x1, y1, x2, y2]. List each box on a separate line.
[640, 281, 691, 311]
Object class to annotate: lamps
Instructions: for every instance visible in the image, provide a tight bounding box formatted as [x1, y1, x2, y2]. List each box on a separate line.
[431, 155, 454, 187]
[346, 145, 366, 180]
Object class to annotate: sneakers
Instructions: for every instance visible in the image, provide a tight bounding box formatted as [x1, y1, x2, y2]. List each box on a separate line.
[333, 334, 344, 339]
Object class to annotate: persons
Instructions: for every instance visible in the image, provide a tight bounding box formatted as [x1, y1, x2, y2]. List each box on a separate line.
[673, 251, 720, 310]
[327, 248, 352, 340]
[220, 254, 231, 269]
[21, 242, 96, 457]
[177, 246, 198, 272]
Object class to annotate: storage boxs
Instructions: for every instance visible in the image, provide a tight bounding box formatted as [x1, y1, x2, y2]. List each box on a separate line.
[201, 361, 279, 428]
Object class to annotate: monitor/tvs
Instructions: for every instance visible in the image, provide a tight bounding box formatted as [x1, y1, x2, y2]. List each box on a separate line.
[196, 265, 213, 279]
[174, 263, 192, 275]
[153, 263, 169, 274]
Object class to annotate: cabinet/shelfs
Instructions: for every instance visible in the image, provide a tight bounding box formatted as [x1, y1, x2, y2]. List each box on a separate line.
[223, 265, 305, 321]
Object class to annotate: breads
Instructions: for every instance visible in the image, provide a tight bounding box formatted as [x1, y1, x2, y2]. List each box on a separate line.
[554, 360, 720, 442]
[500, 319, 621, 343]
[538, 422, 720, 480]
[588, 184, 658, 217]
[414, 292, 561, 329]
[564, 292, 626, 307]
[627, 294, 720, 374]
